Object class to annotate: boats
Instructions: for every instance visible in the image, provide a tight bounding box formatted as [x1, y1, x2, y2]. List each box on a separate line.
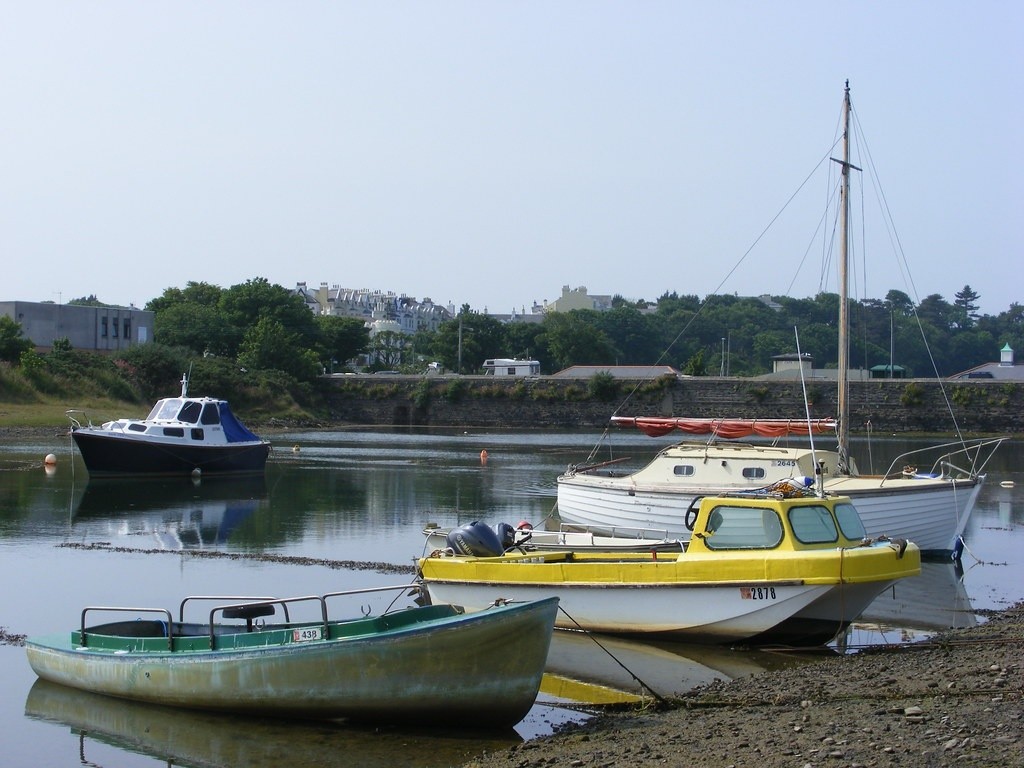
[22, 678, 525, 768]
[64, 362, 272, 474]
[409, 476, 925, 646]
[534, 626, 853, 712]
[18, 582, 563, 723]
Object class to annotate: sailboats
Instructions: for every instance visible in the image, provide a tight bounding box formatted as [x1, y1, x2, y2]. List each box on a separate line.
[848, 556, 983, 633]
[551, 80, 1012, 558]
